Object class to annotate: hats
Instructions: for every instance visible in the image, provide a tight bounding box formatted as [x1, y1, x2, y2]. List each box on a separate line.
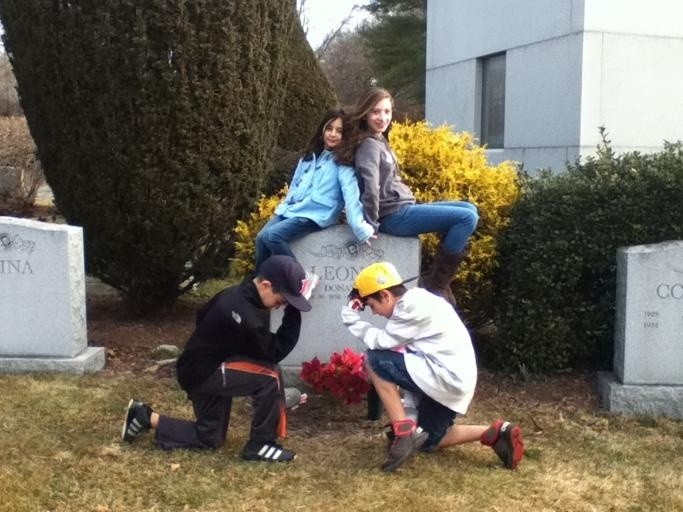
[261, 254, 312, 312]
[355, 261, 418, 298]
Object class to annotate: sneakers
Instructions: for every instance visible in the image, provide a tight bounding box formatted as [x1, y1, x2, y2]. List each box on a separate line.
[481, 418, 522, 468]
[381, 420, 429, 472]
[122, 397, 152, 444]
[242, 439, 298, 463]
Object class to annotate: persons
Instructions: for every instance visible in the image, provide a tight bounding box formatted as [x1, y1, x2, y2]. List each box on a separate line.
[339, 261, 524, 475]
[249, 108, 379, 305]
[330, 85, 479, 310]
[118, 253, 313, 465]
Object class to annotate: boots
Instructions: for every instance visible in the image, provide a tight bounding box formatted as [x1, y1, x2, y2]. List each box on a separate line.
[420, 242, 472, 308]
